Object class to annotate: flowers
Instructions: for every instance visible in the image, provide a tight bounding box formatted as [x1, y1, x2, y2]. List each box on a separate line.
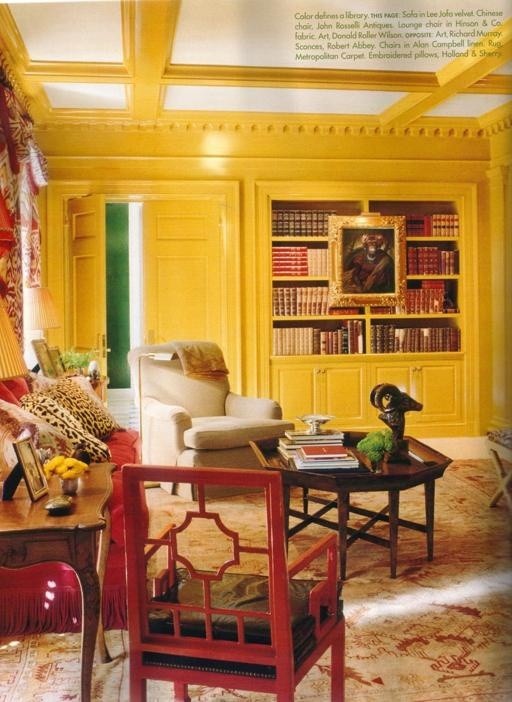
[45, 453, 88, 479]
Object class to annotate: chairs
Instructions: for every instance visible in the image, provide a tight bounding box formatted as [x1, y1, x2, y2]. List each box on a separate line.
[121, 464, 346, 702]
[126, 341, 300, 500]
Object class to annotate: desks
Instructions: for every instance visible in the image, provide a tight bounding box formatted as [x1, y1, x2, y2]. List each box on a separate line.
[0, 460, 118, 702]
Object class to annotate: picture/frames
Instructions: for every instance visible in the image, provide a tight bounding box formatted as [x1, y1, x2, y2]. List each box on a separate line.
[48, 346, 67, 375]
[327, 215, 407, 308]
[30, 338, 57, 379]
[12, 435, 50, 502]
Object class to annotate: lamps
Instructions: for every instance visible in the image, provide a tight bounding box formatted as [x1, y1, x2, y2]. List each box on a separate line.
[136, 351, 179, 490]
[29, 285, 63, 339]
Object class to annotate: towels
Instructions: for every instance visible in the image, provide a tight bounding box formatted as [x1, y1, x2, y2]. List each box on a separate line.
[170, 342, 230, 382]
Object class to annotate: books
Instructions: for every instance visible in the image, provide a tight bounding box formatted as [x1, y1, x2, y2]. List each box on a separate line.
[277, 429, 358, 471]
[271, 209, 459, 355]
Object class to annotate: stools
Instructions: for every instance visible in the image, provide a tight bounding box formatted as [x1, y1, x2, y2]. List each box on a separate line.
[484, 427, 512, 515]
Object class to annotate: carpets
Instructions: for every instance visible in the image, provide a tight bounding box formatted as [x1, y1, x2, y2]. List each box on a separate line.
[0, 446, 511, 701]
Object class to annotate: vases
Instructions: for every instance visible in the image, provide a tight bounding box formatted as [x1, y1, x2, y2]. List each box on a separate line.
[59, 478, 80, 494]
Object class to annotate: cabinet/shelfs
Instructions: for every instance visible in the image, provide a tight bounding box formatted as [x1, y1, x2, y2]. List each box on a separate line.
[269, 195, 465, 358]
[369, 359, 469, 437]
[269, 358, 368, 433]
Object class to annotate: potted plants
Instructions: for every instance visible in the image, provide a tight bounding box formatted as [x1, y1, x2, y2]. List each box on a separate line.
[354, 426, 397, 474]
[60, 350, 90, 377]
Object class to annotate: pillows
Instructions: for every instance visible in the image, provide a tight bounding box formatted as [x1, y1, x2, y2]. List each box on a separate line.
[0, 375, 131, 469]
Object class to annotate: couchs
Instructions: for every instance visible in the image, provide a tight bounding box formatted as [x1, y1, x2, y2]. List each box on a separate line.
[0, 375, 155, 638]
